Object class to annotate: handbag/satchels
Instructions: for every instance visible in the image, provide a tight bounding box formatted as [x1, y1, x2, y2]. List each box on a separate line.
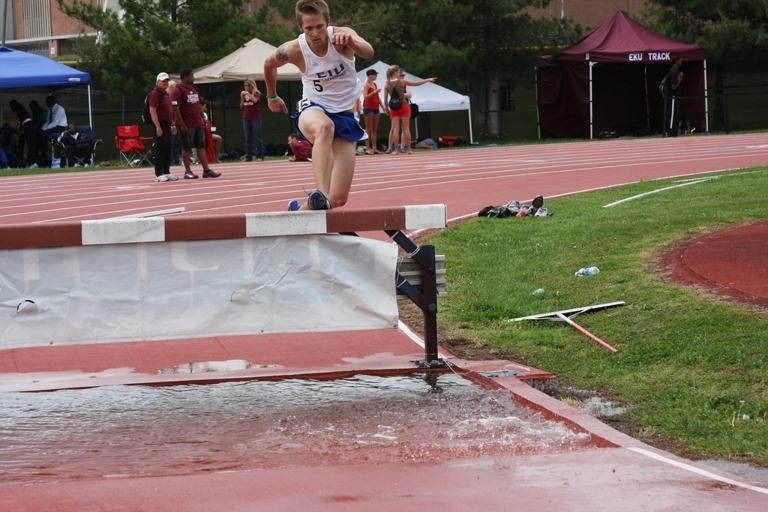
[409, 103, 419, 119]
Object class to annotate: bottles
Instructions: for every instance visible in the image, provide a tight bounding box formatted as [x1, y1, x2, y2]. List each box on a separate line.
[574, 267, 600, 277]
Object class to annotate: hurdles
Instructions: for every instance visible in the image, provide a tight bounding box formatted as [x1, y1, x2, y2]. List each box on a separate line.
[0, 203, 448, 394]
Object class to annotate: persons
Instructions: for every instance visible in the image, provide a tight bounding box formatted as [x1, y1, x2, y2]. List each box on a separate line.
[660, 56, 691, 135]
[384, 65, 439, 155]
[201, 96, 223, 163]
[240, 80, 266, 162]
[388, 72, 411, 152]
[171, 68, 221, 179]
[147, 72, 179, 182]
[29, 95, 67, 168]
[63, 124, 93, 167]
[264, 0, 374, 212]
[164, 79, 183, 166]
[286, 133, 312, 162]
[26, 98, 45, 167]
[363, 69, 388, 156]
[9, 99, 32, 160]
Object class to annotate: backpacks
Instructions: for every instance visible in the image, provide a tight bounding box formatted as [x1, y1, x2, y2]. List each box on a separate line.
[387, 80, 403, 110]
[142, 88, 162, 124]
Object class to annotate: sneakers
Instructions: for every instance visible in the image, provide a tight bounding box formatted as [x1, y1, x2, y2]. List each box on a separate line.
[365, 146, 413, 154]
[287, 200, 302, 211]
[184, 171, 199, 179]
[203, 169, 222, 178]
[308, 189, 330, 210]
[158, 173, 178, 182]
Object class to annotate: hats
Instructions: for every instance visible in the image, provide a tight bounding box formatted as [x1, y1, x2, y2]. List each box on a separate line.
[367, 69, 378, 76]
[157, 72, 170, 81]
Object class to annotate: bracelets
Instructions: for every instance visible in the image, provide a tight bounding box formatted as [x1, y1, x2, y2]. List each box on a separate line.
[266, 95, 279, 101]
[170, 124, 176, 128]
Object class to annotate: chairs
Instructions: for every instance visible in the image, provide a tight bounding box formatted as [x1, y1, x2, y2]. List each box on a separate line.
[54, 127, 105, 169]
[113, 122, 155, 169]
